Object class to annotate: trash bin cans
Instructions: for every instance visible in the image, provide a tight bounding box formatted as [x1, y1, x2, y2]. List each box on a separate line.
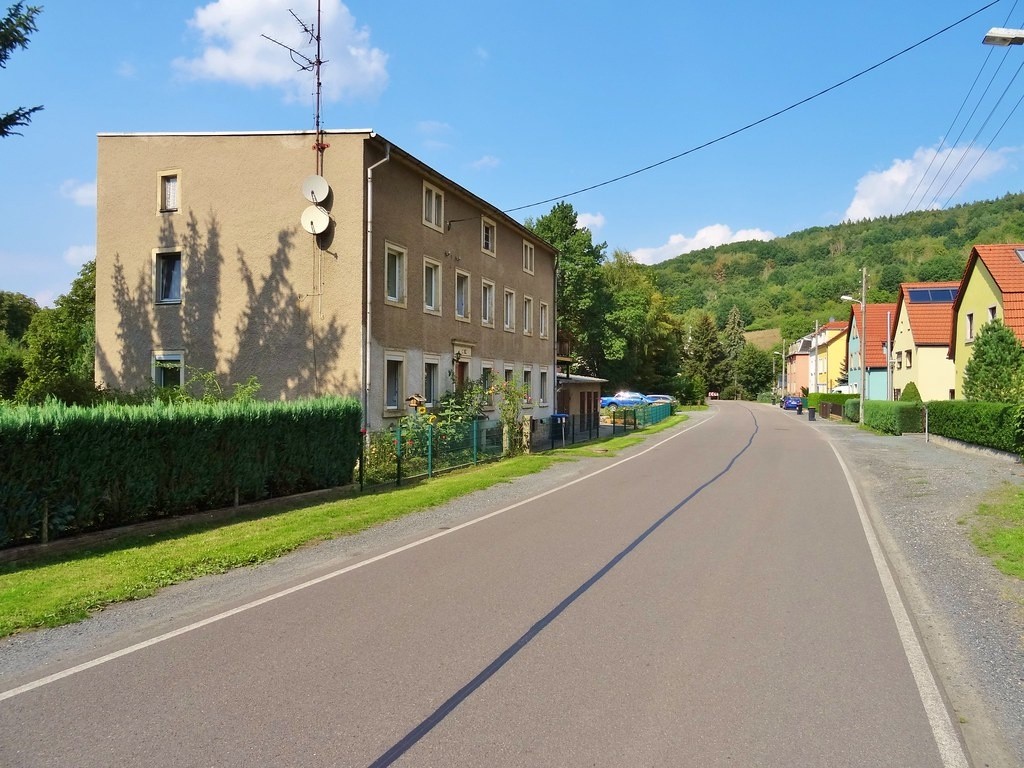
[771, 398, 775, 405]
[551, 414, 569, 440]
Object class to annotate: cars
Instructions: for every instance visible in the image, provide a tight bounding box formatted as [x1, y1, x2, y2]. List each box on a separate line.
[600, 392, 654, 413]
[780, 395, 802, 410]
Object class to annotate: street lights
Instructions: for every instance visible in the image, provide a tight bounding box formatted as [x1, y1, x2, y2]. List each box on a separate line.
[774, 339, 785, 401]
[840, 268, 870, 427]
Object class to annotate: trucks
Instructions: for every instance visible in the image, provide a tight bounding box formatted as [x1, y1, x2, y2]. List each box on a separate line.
[832, 386, 849, 394]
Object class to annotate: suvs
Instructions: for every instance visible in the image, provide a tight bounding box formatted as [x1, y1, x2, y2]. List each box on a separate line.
[646, 395, 679, 403]
